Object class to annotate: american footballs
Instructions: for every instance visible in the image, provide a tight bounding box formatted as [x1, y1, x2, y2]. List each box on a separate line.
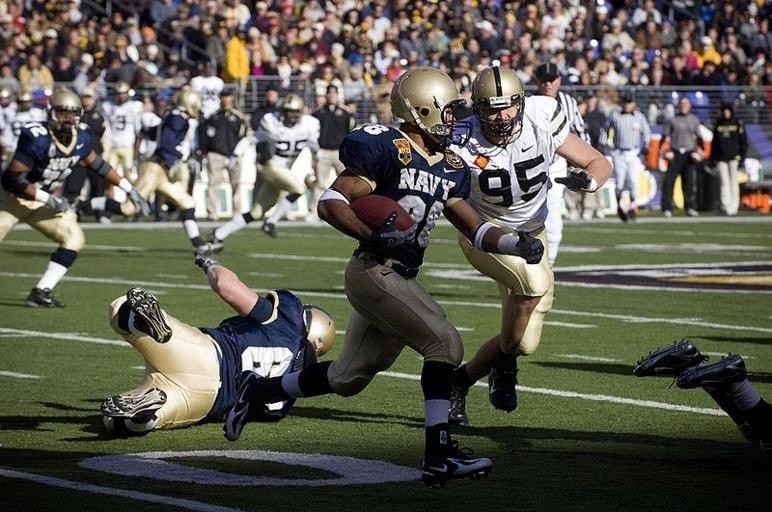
[348, 194, 416, 232]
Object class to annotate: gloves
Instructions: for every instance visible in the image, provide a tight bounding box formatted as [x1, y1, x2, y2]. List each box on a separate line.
[44, 194, 69, 213]
[551, 162, 602, 201]
[512, 232, 548, 266]
[126, 187, 152, 216]
[190, 253, 223, 278]
[364, 209, 422, 257]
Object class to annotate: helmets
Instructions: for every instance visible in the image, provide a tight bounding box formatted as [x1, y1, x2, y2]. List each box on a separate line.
[111, 80, 130, 92]
[389, 66, 475, 151]
[47, 89, 85, 135]
[19, 91, 32, 101]
[280, 93, 306, 126]
[469, 66, 526, 148]
[176, 92, 202, 118]
[0, 87, 11, 99]
[299, 302, 336, 359]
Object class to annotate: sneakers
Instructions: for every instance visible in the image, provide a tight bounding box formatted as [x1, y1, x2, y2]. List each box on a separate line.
[675, 354, 747, 391]
[685, 207, 701, 217]
[631, 338, 704, 377]
[203, 233, 226, 253]
[99, 386, 168, 422]
[660, 208, 672, 216]
[191, 241, 217, 256]
[258, 222, 280, 240]
[419, 442, 494, 491]
[25, 287, 66, 310]
[124, 284, 175, 345]
[221, 369, 270, 443]
[486, 352, 519, 412]
[446, 367, 473, 429]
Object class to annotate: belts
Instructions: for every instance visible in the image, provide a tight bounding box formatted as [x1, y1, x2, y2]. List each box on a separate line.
[615, 145, 639, 153]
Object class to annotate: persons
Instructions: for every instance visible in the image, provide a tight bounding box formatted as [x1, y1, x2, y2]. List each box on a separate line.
[615, 1, 767, 87]
[599, 96, 651, 223]
[529, 63, 591, 266]
[220, 67, 546, 487]
[148, 0, 219, 90]
[0, 88, 151, 309]
[99, 247, 340, 437]
[251, 88, 281, 132]
[100, 0, 145, 78]
[204, 93, 322, 254]
[195, 87, 250, 218]
[0, 0, 97, 86]
[69, 91, 224, 254]
[220, 0, 615, 81]
[445, 67, 615, 428]
[98, 81, 145, 217]
[656, 96, 705, 218]
[5, 92, 48, 172]
[304, 84, 357, 223]
[633, 338, 772, 458]
[708, 101, 749, 217]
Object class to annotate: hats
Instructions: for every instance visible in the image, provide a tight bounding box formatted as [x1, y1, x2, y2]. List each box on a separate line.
[325, 84, 340, 97]
[475, 21, 497, 36]
[262, 84, 279, 90]
[78, 88, 95, 97]
[219, 87, 234, 98]
[584, 90, 597, 98]
[535, 64, 560, 81]
